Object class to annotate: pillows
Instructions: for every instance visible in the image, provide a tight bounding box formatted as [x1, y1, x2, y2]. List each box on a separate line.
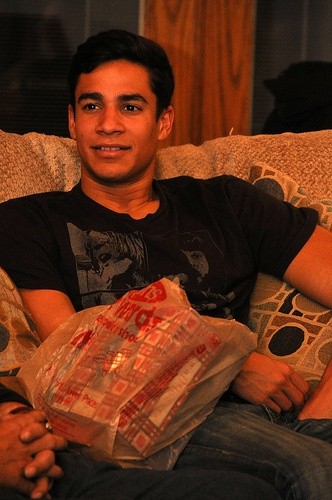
[235, 160, 332, 415]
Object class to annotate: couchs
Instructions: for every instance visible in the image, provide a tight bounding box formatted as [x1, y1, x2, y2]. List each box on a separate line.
[0, 129, 332, 444]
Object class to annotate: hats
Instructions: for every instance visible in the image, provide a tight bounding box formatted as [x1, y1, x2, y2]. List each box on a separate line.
[262, 62, 332, 109]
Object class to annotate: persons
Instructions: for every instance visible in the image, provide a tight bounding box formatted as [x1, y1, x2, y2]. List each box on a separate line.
[0, 269, 283, 500]
[0, 28, 332, 500]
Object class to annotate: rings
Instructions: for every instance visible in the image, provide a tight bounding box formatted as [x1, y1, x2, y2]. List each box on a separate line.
[39, 419, 54, 433]
